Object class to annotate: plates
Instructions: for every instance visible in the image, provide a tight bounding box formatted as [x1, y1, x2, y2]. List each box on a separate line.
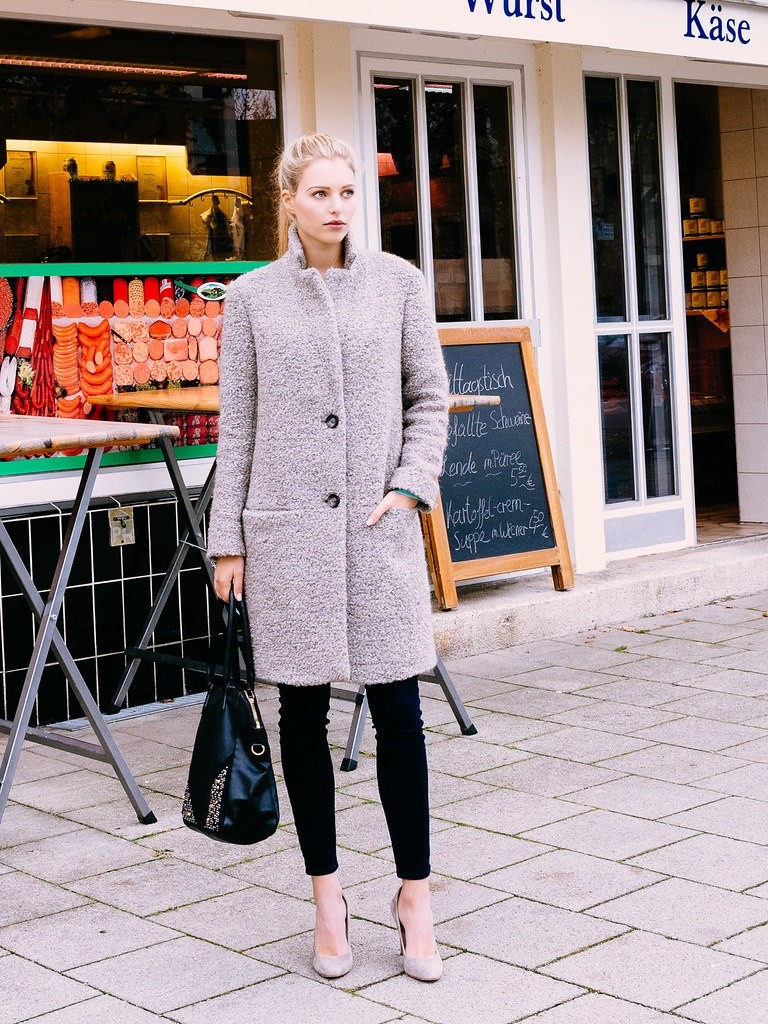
[197, 282, 227, 300]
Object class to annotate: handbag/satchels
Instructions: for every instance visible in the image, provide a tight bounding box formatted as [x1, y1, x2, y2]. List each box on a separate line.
[181, 591, 279, 845]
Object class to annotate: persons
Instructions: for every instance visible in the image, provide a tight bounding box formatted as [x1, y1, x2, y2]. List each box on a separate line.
[206, 134, 451, 980]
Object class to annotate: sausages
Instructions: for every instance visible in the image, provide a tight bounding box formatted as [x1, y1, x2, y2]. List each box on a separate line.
[0, 276, 116, 457]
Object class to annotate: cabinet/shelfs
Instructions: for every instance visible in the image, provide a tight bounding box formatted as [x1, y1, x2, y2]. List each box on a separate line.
[683, 234, 731, 439]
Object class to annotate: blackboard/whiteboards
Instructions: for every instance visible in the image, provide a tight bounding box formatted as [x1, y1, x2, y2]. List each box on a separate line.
[420, 325, 570, 582]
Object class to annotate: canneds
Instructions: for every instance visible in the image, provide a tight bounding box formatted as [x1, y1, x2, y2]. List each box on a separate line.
[685, 251, 729, 311]
[682, 194, 725, 237]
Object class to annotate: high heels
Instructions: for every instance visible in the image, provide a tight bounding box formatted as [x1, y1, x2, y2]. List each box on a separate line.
[313, 893, 353, 979]
[390, 885, 442, 982]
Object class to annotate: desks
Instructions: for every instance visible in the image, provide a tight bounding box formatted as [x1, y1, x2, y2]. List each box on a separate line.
[0, 414, 180, 827]
[85, 385, 503, 771]
